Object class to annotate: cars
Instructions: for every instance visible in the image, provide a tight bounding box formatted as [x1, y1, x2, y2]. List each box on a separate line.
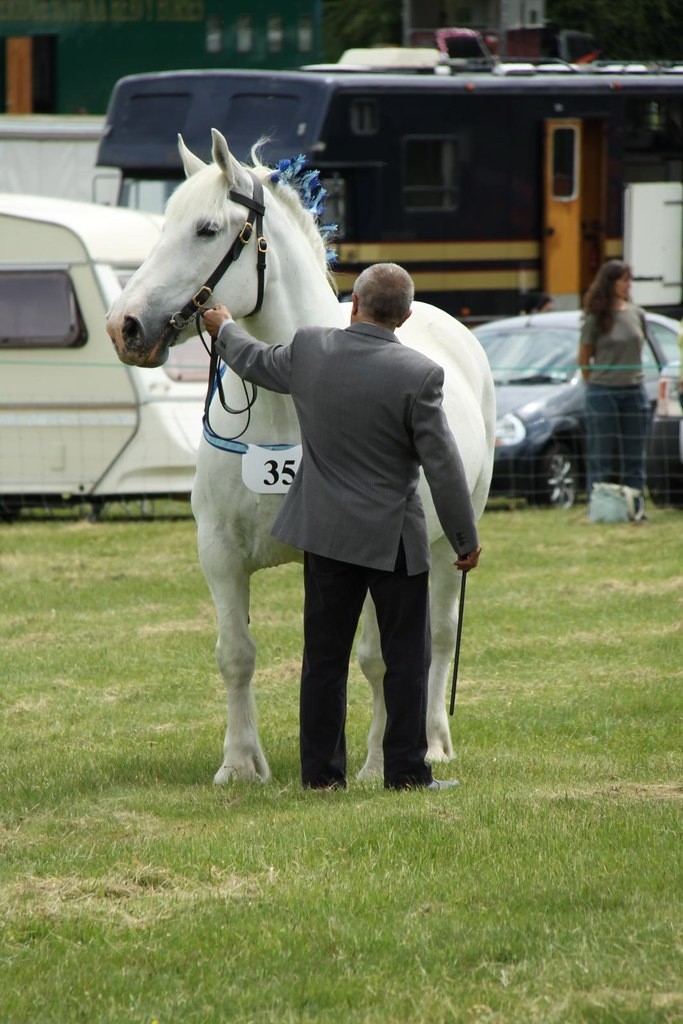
[469, 307, 683, 508]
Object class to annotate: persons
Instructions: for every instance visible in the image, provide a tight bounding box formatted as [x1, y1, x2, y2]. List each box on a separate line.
[203, 262, 482, 793]
[579, 261, 668, 522]
[519, 289, 554, 315]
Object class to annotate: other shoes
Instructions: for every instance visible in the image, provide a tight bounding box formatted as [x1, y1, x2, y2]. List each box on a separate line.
[428, 778, 459, 790]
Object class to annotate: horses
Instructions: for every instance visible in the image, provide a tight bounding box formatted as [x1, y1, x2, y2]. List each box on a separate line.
[107, 129, 498, 786]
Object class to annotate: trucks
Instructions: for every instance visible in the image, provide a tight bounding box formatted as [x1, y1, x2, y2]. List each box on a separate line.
[95, 28, 682, 324]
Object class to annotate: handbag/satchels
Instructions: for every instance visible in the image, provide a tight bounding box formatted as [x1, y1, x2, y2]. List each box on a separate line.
[589, 481, 645, 524]
